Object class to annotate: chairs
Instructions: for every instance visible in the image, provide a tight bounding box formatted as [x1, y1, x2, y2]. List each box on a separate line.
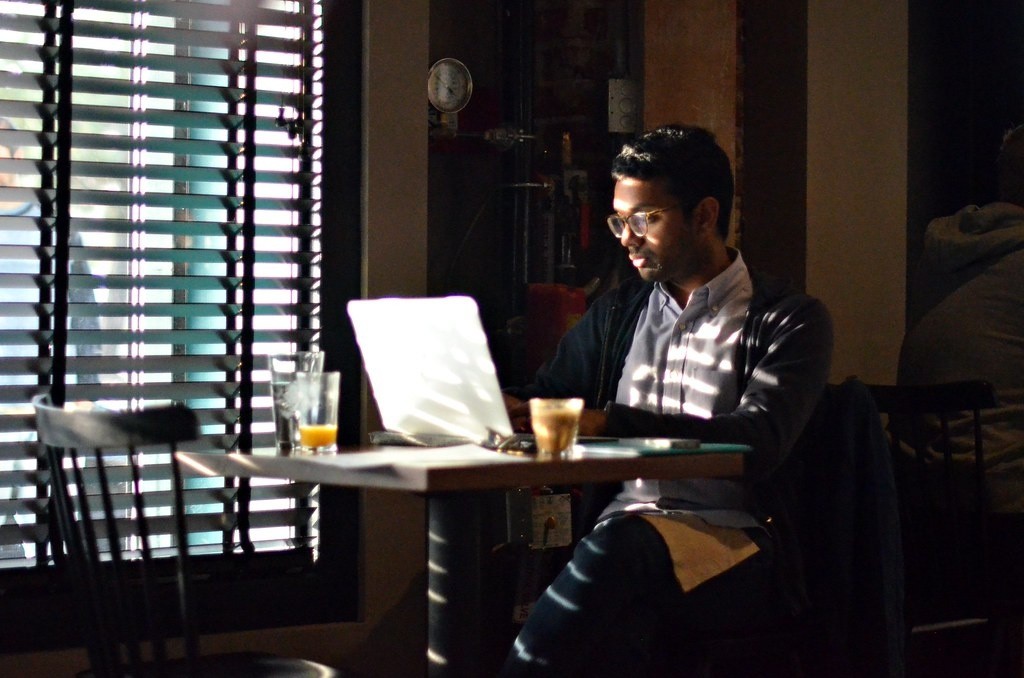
[32, 393, 343, 678]
[648, 371, 908, 678]
[866, 379, 1024, 678]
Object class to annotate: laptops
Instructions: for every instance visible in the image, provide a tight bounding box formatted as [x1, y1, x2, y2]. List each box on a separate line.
[346, 296, 619, 444]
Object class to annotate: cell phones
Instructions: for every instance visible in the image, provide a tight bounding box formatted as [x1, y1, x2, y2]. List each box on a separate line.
[644, 437, 699, 449]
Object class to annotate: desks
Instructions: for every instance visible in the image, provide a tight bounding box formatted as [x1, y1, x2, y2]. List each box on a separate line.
[175, 434, 744, 678]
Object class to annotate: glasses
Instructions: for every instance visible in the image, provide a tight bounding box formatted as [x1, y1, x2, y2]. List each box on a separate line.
[606, 204, 675, 239]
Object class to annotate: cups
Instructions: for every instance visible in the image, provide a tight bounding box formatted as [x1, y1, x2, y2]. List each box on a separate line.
[268, 352, 326, 448]
[296, 371, 339, 456]
[529, 396, 584, 459]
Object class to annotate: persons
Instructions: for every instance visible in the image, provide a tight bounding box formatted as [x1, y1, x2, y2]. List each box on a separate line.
[898, 122, 1023, 601]
[502, 122, 836, 678]
